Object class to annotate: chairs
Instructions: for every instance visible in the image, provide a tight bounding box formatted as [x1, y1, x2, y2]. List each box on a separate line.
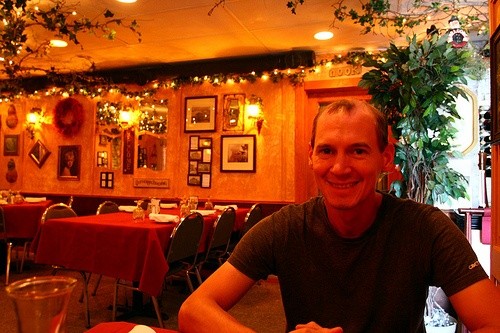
[2, 200, 264, 328]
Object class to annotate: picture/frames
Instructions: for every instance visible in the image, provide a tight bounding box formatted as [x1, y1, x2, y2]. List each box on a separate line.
[29, 140, 51, 167]
[220, 135, 257, 174]
[185, 95, 216, 133]
[4, 135, 20, 156]
[58, 145, 81, 180]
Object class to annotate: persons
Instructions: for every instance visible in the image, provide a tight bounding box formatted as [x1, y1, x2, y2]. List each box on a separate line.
[177, 96, 500, 333]
[60, 151, 76, 177]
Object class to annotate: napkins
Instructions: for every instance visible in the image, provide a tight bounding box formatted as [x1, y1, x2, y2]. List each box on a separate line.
[148, 214, 180, 223]
[189, 209, 216, 215]
[160, 203, 178, 208]
[118, 206, 139, 212]
[214, 205, 238, 211]
[26, 197, 46, 203]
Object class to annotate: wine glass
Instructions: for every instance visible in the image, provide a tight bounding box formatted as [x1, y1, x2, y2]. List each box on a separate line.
[204, 195, 214, 210]
[133, 200, 145, 223]
[177, 197, 190, 217]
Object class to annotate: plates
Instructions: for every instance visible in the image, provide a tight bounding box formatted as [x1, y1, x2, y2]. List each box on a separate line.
[124, 207, 231, 223]
[27, 201, 42, 202]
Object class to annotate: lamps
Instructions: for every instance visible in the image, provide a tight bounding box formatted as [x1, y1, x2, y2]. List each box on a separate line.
[26, 108, 43, 138]
[119, 105, 132, 126]
[247, 95, 261, 118]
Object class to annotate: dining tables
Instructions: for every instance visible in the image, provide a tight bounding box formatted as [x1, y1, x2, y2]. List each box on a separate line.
[32, 207, 250, 327]
[0, 198, 52, 239]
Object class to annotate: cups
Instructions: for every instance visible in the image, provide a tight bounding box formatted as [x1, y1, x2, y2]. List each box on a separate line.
[5, 276, 78, 333]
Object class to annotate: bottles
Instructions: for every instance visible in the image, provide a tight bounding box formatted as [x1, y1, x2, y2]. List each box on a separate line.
[1, 189, 24, 204]
[187, 199, 197, 209]
[146, 203, 158, 214]
